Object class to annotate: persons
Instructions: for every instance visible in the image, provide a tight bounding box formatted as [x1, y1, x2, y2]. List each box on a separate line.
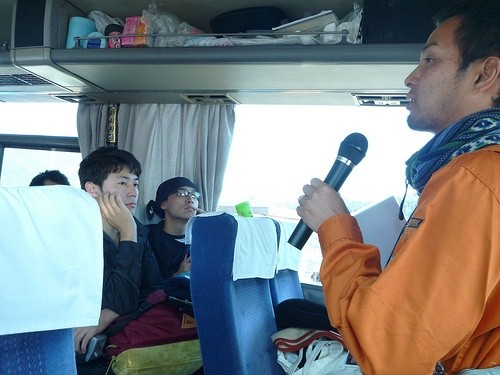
[28, 171, 71, 186]
[296, 5, 500, 374]
[145, 177, 211, 304]
[74, 147, 161, 375]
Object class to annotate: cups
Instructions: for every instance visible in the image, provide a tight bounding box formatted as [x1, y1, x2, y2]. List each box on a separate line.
[235, 201, 253, 218]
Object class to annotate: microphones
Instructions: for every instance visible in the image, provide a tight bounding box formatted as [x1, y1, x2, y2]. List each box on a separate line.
[287, 132, 368, 250]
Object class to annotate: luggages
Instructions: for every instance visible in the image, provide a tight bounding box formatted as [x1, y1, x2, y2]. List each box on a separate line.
[209, 6, 290, 39]
[356, 0, 438, 45]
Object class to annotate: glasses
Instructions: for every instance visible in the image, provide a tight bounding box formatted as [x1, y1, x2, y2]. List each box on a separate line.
[167, 189, 201, 200]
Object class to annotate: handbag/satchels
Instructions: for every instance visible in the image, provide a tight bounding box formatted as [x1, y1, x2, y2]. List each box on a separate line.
[111, 339, 204, 375]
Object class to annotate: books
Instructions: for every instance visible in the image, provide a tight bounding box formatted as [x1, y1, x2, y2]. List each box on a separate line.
[271, 9, 338, 38]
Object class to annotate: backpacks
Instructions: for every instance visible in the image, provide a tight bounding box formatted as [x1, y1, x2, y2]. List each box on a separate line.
[102, 289, 199, 357]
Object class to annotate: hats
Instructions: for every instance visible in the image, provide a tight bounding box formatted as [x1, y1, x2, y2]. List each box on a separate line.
[163, 276, 195, 320]
[154, 177, 198, 217]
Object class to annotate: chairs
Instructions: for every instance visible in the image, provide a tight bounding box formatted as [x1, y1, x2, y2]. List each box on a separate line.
[0, 185, 104, 375]
[189, 213, 308, 375]
